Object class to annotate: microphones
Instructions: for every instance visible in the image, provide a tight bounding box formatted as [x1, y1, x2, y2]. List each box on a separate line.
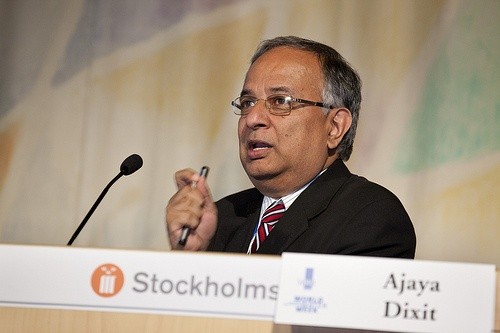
[67, 154, 143, 245]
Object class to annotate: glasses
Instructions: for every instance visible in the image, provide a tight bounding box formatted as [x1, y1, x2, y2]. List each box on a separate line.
[232, 94, 334, 116]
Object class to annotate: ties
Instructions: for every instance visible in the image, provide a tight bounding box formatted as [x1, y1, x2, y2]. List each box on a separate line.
[251, 201, 286, 254]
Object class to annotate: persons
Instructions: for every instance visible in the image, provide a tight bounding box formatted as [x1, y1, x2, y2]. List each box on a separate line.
[165, 36, 416, 261]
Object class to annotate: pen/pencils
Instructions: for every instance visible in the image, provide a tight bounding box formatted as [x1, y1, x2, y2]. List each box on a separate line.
[177, 166, 209, 249]
